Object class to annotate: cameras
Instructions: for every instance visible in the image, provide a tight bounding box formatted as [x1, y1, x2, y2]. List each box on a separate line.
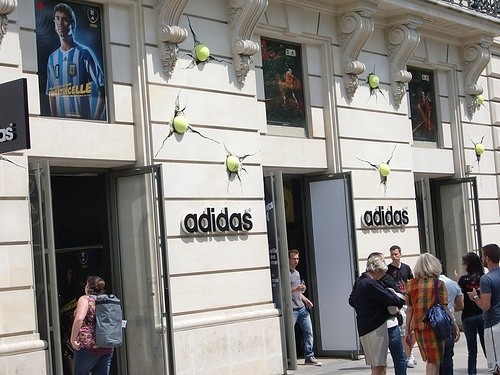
[471, 288, 480, 296]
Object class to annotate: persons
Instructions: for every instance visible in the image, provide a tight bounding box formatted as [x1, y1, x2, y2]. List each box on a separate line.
[405, 253, 460, 375]
[282, 68, 301, 112]
[45, 3, 106, 119]
[69, 275, 115, 375]
[288, 250, 322, 365]
[454, 252, 487, 375]
[418, 90, 432, 132]
[467, 244, 500, 375]
[438, 258, 464, 375]
[348, 245, 417, 375]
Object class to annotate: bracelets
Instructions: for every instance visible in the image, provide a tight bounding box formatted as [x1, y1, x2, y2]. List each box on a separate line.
[473, 295, 478, 301]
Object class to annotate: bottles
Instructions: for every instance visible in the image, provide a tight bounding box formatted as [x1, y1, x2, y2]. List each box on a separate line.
[300, 279, 306, 293]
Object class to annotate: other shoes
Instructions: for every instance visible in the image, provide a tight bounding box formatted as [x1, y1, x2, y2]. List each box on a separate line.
[411, 355, 417, 365]
[305, 357, 322, 365]
[407, 359, 414, 368]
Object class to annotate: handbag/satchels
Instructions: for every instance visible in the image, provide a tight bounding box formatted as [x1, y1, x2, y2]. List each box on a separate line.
[422, 304, 451, 340]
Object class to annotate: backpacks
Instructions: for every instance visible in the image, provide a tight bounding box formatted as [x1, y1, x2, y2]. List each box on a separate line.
[86, 294, 122, 347]
